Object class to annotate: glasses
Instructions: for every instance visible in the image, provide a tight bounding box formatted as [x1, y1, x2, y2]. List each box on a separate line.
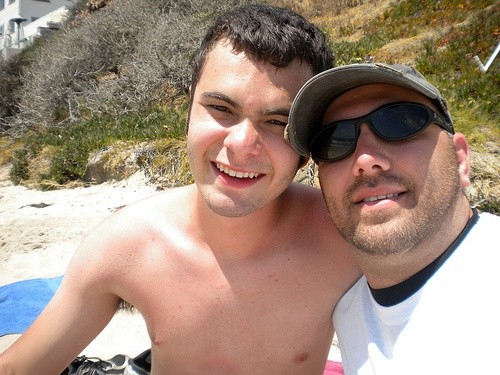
[306, 99, 455, 163]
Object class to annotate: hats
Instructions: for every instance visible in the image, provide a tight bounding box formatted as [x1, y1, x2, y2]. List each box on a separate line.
[284, 62, 456, 158]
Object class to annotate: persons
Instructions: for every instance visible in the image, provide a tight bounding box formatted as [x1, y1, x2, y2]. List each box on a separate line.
[1, 6, 366, 374]
[281, 62, 500, 375]
